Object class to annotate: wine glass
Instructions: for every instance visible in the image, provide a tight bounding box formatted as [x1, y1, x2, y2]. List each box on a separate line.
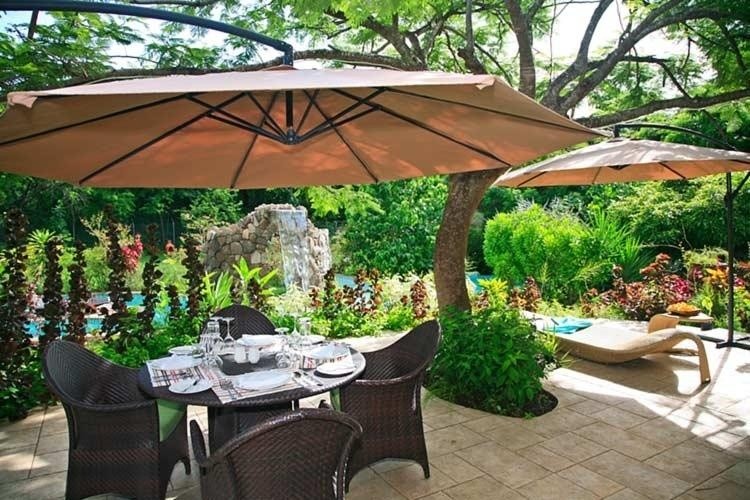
[199, 316, 237, 374]
[276, 312, 312, 367]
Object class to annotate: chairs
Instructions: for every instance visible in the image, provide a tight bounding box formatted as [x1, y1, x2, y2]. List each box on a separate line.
[202, 303, 281, 339]
[337, 318, 446, 496]
[190, 406, 363, 500]
[40, 335, 193, 499]
[516, 310, 715, 383]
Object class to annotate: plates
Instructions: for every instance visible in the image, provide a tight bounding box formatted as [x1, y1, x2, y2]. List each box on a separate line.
[151, 357, 203, 370]
[302, 345, 349, 359]
[168, 347, 197, 355]
[167, 380, 213, 395]
[667, 310, 700, 316]
[237, 335, 273, 345]
[316, 363, 356, 377]
[307, 334, 325, 343]
[231, 369, 293, 391]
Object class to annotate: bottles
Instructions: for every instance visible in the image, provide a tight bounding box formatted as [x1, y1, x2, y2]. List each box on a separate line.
[249, 346, 260, 364]
[235, 343, 247, 364]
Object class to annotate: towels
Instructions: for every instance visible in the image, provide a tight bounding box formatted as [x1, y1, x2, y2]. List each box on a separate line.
[543, 316, 593, 333]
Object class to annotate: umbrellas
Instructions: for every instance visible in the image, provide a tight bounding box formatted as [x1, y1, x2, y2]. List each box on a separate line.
[494, 122, 750, 343]
[0, 0, 612, 189]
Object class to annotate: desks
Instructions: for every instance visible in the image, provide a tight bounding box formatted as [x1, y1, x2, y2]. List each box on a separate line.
[647, 308, 714, 334]
[136, 339, 366, 456]
